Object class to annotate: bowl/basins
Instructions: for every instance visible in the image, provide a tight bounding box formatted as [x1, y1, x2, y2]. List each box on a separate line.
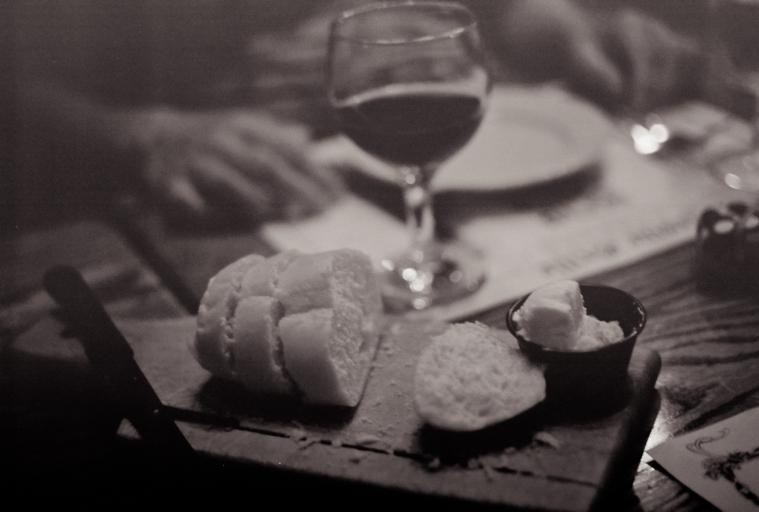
[505, 282, 648, 394]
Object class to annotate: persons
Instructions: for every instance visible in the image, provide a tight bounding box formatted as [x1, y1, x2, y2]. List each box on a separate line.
[0, 1, 709, 229]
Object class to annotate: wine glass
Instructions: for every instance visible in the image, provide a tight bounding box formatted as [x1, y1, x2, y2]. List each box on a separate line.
[329, 5, 491, 307]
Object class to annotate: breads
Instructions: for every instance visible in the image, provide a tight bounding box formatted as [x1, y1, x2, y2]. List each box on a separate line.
[194, 249, 263, 374]
[508, 281, 645, 395]
[278, 251, 383, 410]
[235, 253, 311, 397]
[413, 318, 545, 434]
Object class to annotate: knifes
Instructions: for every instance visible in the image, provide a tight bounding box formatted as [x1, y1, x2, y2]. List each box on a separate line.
[33, 266, 200, 493]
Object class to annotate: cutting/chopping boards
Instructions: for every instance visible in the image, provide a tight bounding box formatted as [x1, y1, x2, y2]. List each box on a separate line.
[32, 270, 662, 512]
